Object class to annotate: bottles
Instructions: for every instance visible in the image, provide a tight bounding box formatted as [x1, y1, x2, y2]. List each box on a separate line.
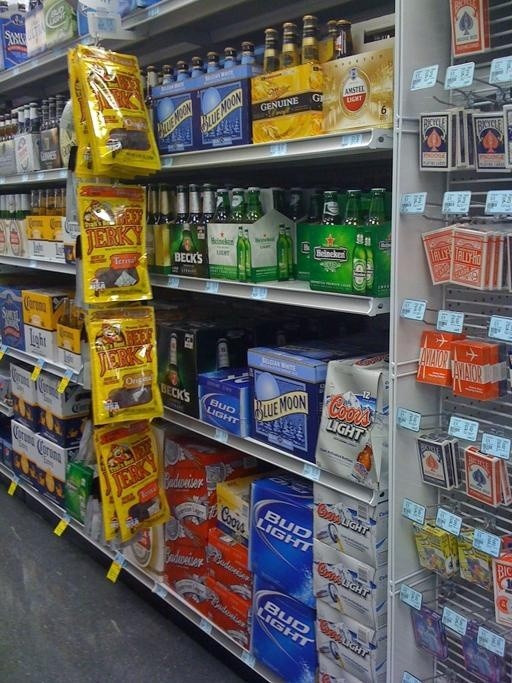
[3, 16, 398, 483]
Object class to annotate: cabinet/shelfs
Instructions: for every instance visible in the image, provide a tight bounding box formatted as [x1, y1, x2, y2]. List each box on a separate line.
[0, 2, 392, 683]
